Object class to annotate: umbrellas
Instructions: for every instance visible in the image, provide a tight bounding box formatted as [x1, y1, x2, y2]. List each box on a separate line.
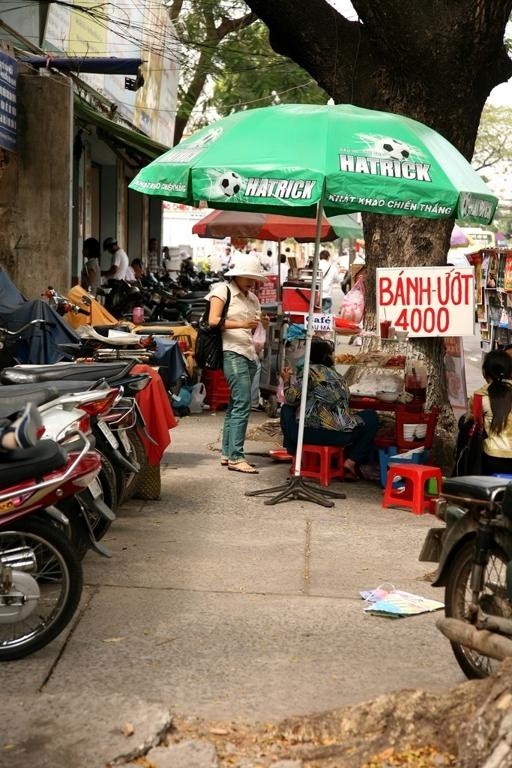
[127, 97, 498, 477]
[189, 210, 362, 300]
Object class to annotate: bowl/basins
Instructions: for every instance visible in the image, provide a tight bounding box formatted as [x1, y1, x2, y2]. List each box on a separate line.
[395, 331, 409, 337]
[376, 392, 398, 403]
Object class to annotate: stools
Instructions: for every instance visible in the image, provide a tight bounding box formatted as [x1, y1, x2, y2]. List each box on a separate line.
[393, 403, 440, 450]
[287, 443, 346, 489]
[378, 445, 428, 490]
[201, 368, 230, 412]
[380, 462, 443, 516]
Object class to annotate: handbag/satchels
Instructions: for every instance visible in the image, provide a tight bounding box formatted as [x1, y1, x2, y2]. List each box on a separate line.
[464, 395, 486, 452]
[196, 324, 224, 372]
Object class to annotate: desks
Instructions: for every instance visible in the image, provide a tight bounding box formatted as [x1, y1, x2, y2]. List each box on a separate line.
[337, 393, 425, 452]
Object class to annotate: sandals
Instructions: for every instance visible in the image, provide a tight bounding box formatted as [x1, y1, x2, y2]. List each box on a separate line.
[227, 461, 258, 474]
[221, 458, 256, 467]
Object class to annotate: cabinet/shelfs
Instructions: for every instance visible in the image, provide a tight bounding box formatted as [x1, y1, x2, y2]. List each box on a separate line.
[332, 331, 408, 400]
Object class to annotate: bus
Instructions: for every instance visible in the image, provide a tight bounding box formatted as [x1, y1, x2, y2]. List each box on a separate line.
[445, 228, 498, 266]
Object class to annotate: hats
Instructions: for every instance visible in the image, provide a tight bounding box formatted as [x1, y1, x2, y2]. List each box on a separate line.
[223, 254, 267, 280]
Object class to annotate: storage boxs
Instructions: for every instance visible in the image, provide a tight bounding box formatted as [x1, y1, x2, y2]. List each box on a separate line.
[279, 286, 319, 324]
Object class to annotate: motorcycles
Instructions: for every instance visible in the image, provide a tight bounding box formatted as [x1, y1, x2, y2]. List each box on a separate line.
[416, 471, 512, 680]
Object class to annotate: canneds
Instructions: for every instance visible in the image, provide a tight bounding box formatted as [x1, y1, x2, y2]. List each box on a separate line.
[132, 307, 144, 324]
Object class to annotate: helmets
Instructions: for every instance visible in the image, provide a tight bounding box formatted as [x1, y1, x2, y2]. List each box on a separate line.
[103, 237, 117, 252]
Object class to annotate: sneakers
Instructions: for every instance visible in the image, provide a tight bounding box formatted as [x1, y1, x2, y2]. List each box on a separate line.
[345, 468, 360, 483]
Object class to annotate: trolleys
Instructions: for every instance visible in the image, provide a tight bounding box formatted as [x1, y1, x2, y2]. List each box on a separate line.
[253, 309, 338, 419]
